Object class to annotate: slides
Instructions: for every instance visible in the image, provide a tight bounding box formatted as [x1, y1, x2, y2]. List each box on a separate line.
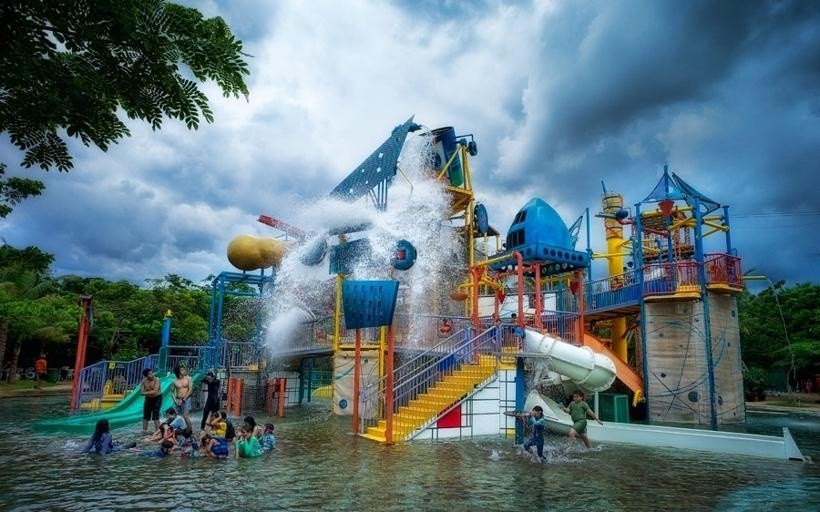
[34, 362, 208, 438]
[523, 328, 806, 464]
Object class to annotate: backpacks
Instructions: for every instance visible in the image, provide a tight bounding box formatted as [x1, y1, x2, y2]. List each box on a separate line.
[220, 420, 236, 440]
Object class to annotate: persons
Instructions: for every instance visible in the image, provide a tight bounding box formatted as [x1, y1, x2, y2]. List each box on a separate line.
[626, 261, 634, 281]
[560, 390, 604, 448]
[112, 373, 126, 393]
[624, 266, 628, 280]
[517, 402, 547, 463]
[34, 353, 48, 389]
[199, 368, 221, 431]
[307, 311, 541, 365]
[140, 368, 163, 432]
[80, 419, 137, 456]
[171, 365, 193, 431]
[128, 405, 281, 462]
